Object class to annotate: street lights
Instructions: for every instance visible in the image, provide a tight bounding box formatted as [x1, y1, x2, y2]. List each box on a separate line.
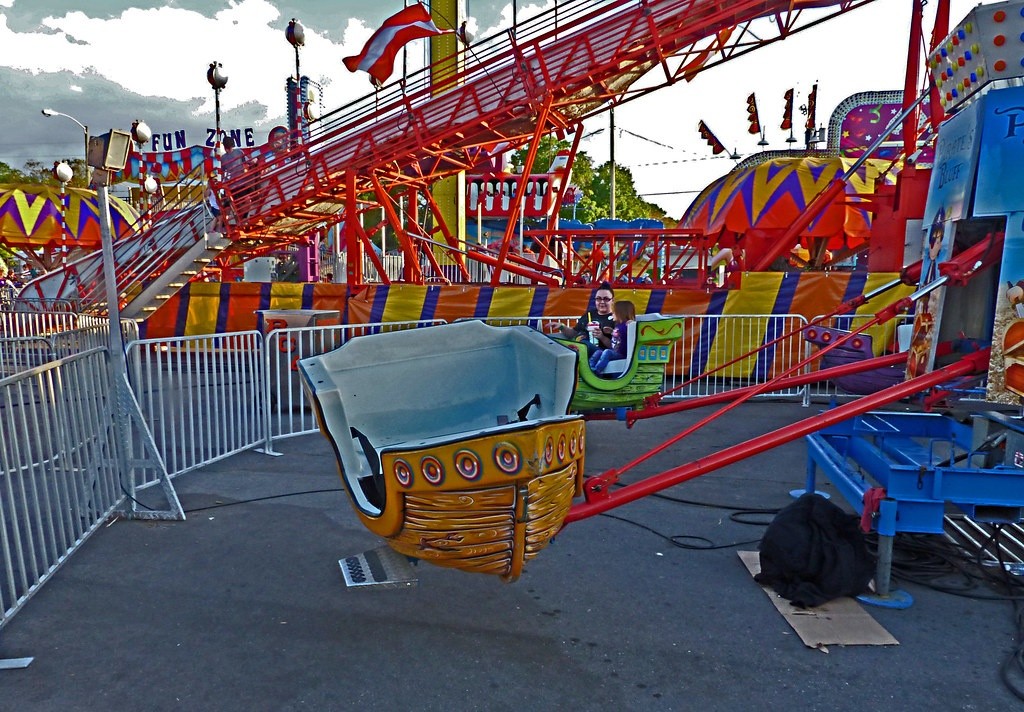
[40, 108, 90, 189]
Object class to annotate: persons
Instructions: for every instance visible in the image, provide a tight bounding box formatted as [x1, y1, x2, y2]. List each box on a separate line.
[475, 242, 653, 286]
[0, 263, 32, 288]
[545, 280, 636, 375]
[203, 136, 256, 232]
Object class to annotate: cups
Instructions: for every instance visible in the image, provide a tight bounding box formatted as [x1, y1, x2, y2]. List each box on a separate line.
[588, 321, 599, 347]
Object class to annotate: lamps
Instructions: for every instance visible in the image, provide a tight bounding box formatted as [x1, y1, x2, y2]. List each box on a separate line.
[786, 122, 797, 142]
[757, 125, 769, 145]
[731, 148, 741, 160]
[808, 132, 821, 143]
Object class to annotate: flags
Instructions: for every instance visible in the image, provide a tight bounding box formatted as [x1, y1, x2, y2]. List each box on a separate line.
[342, 3, 457, 87]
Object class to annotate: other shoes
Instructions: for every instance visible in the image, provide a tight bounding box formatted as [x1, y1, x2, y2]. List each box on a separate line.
[592, 368, 603, 378]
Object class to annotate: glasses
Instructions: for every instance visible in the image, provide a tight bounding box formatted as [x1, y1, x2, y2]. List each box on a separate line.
[595, 297, 613, 302]
